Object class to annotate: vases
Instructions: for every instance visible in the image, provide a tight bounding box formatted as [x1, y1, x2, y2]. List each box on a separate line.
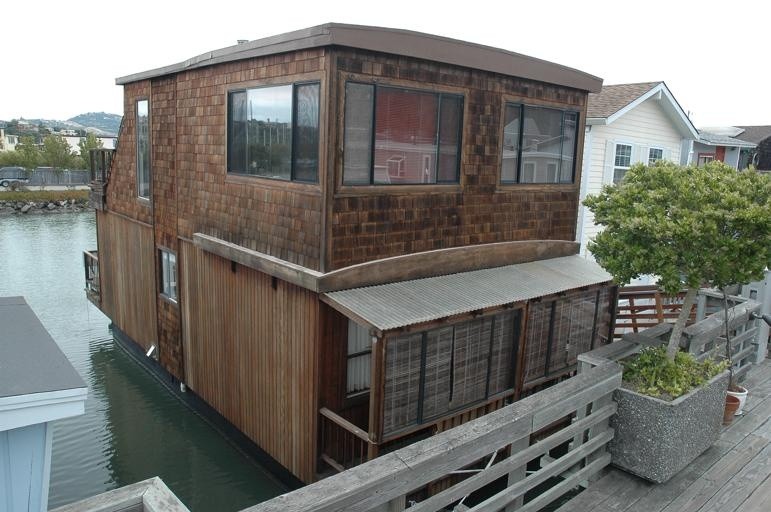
[722, 396, 739, 426]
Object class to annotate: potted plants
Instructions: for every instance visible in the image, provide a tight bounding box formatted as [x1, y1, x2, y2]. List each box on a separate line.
[581, 160, 770, 485]
[721, 285, 748, 417]
